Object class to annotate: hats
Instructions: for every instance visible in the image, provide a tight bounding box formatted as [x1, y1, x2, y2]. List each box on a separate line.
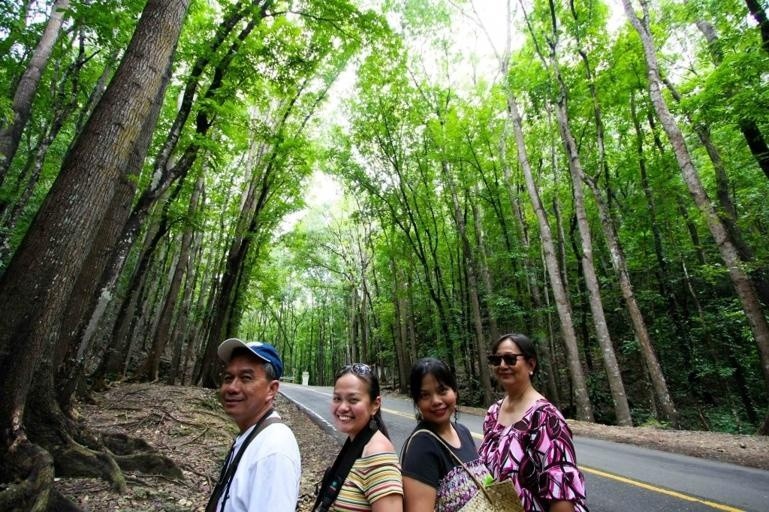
[217, 337, 283, 378]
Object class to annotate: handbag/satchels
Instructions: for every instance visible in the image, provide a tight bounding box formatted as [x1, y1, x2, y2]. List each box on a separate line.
[457, 477, 528, 512]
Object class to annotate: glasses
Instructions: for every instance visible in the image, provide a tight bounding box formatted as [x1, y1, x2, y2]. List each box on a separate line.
[486, 353, 523, 365]
[335, 362, 371, 378]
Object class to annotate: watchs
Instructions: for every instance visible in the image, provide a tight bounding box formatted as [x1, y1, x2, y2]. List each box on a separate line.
[400, 357, 495, 511]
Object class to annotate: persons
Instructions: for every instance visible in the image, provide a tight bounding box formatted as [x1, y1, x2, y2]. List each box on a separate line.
[478, 332, 590, 511]
[310, 362, 405, 511]
[206, 338, 302, 511]
